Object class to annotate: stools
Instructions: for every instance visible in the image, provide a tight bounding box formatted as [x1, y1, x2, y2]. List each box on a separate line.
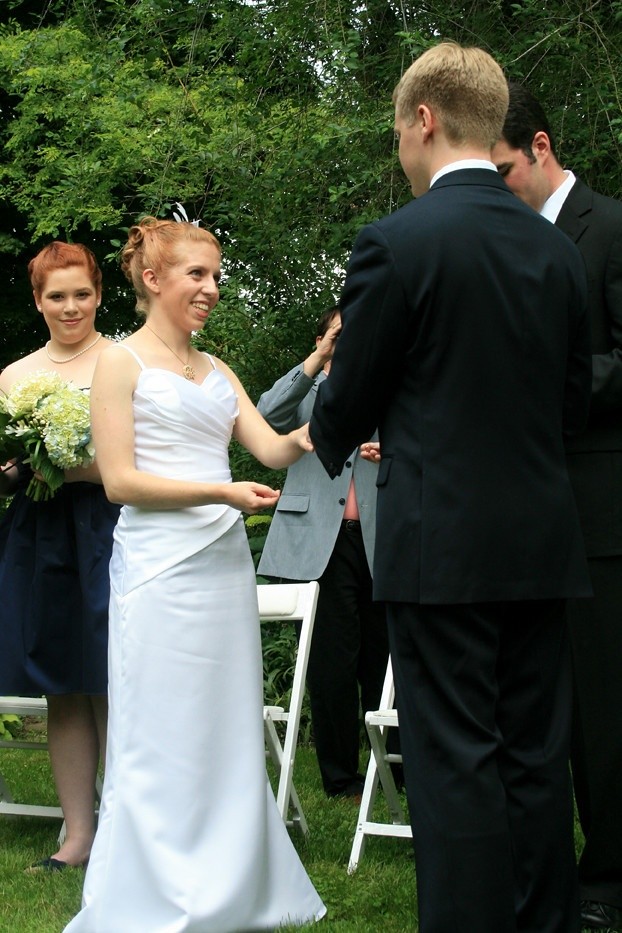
[0, 696, 103, 848]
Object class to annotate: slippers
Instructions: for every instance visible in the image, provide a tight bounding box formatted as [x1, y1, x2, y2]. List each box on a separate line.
[23, 857, 69, 876]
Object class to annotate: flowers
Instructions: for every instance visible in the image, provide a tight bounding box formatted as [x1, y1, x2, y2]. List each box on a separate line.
[0, 369, 93, 504]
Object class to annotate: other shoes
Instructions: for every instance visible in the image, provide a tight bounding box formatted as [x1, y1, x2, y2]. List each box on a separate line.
[579, 902, 620, 927]
[347, 795, 361, 804]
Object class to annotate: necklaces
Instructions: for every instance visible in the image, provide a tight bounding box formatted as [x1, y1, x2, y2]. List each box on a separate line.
[145, 324, 197, 380]
[46, 332, 101, 364]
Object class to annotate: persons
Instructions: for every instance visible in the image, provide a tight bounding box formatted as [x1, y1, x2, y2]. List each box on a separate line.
[307, 42, 622, 933]
[255, 307, 404, 797]
[0, 243, 119, 872]
[64, 217, 328, 933]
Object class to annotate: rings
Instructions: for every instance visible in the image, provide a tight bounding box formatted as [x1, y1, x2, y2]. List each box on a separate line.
[332, 325, 336, 328]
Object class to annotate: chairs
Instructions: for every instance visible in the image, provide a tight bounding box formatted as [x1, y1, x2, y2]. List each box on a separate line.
[346, 652, 413, 878]
[257, 580, 319, 839]
